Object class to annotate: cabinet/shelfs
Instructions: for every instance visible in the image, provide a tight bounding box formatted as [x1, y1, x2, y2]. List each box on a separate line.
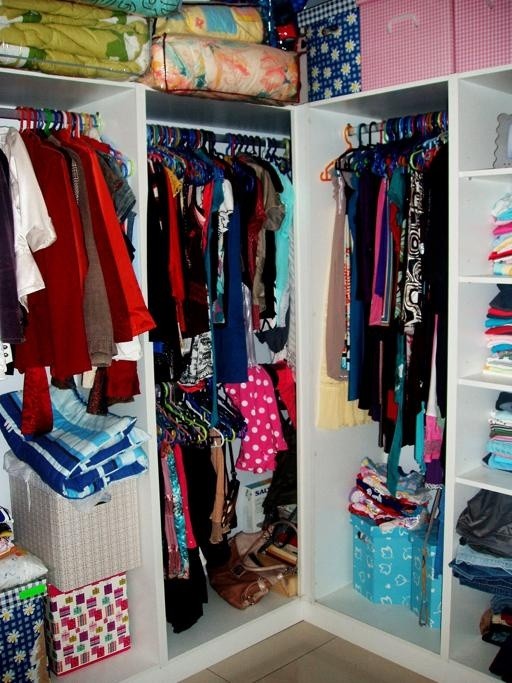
[2, 64, 512, 682]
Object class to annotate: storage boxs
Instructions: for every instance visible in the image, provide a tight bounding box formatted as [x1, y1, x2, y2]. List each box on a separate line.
[1, 580, 48, 683]
[408, 531, 442, 629]
[8, 469, 141, 591]
[43, 572, 131, 679]
[349, 514, 412, 606]
[357, 1, 454, 91]
[297, 0, 361, 102]
[454, 0, 512, 73]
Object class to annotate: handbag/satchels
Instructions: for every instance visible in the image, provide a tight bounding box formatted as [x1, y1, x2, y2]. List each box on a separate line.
[206, 530, 293, 612]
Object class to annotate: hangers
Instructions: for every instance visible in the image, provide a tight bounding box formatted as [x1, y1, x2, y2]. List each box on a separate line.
[156, 381, 246, 448]
[319, 110, 448, 182]
[146, 123, 291, 177]
[16, 106, 134, 178]
